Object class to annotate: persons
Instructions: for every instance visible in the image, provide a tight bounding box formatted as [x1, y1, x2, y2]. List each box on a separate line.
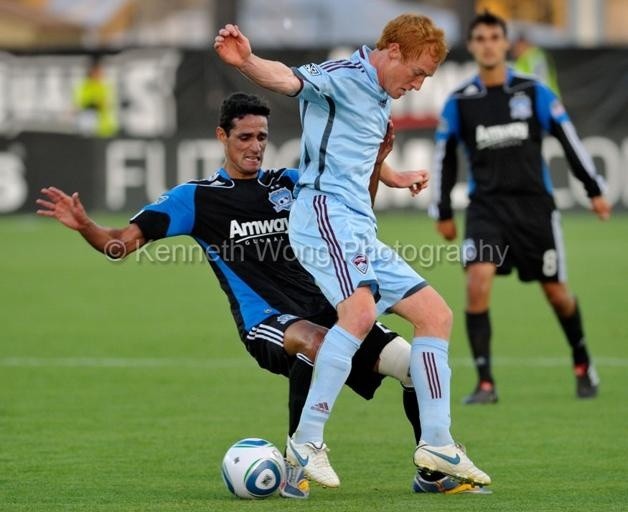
[72, 57, 120, 140]
[425, 13, 611, 405]
[213, 11, 495, 489]
[506, 19, 561, 100]
[35, 92, 494, 498]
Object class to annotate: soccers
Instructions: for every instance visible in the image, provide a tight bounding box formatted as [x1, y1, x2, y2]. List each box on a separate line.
[220, 437, 288, 500]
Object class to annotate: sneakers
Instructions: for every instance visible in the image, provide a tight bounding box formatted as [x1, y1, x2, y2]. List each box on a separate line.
[408, 469, 493, 497]
[279, 444, 313, 501]
[284, 432, 344, 490]
[411, 437, 494, 488]
[572, 353, 601, 398]
[461, 381, 500, 409]
